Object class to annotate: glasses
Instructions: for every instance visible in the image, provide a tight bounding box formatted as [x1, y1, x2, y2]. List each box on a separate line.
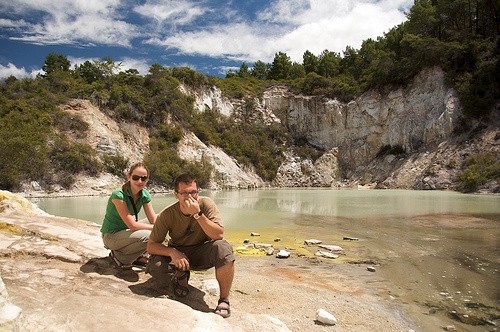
[175, 190, 198, 196]
[131, 175, 148, 181]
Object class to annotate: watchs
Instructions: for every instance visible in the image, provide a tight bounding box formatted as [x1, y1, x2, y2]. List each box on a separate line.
[193, 211, 202, 219]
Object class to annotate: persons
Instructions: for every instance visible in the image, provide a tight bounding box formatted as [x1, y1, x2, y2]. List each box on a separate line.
[100, 163, 159, 269]
[147, 174, 235, 318]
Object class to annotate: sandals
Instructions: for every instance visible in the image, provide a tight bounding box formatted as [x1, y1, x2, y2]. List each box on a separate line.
[109, 250, 132, 271]
[174, 270, 190, 297]
[133, 256, 149, 266]
[214, 298, 230, 318]
